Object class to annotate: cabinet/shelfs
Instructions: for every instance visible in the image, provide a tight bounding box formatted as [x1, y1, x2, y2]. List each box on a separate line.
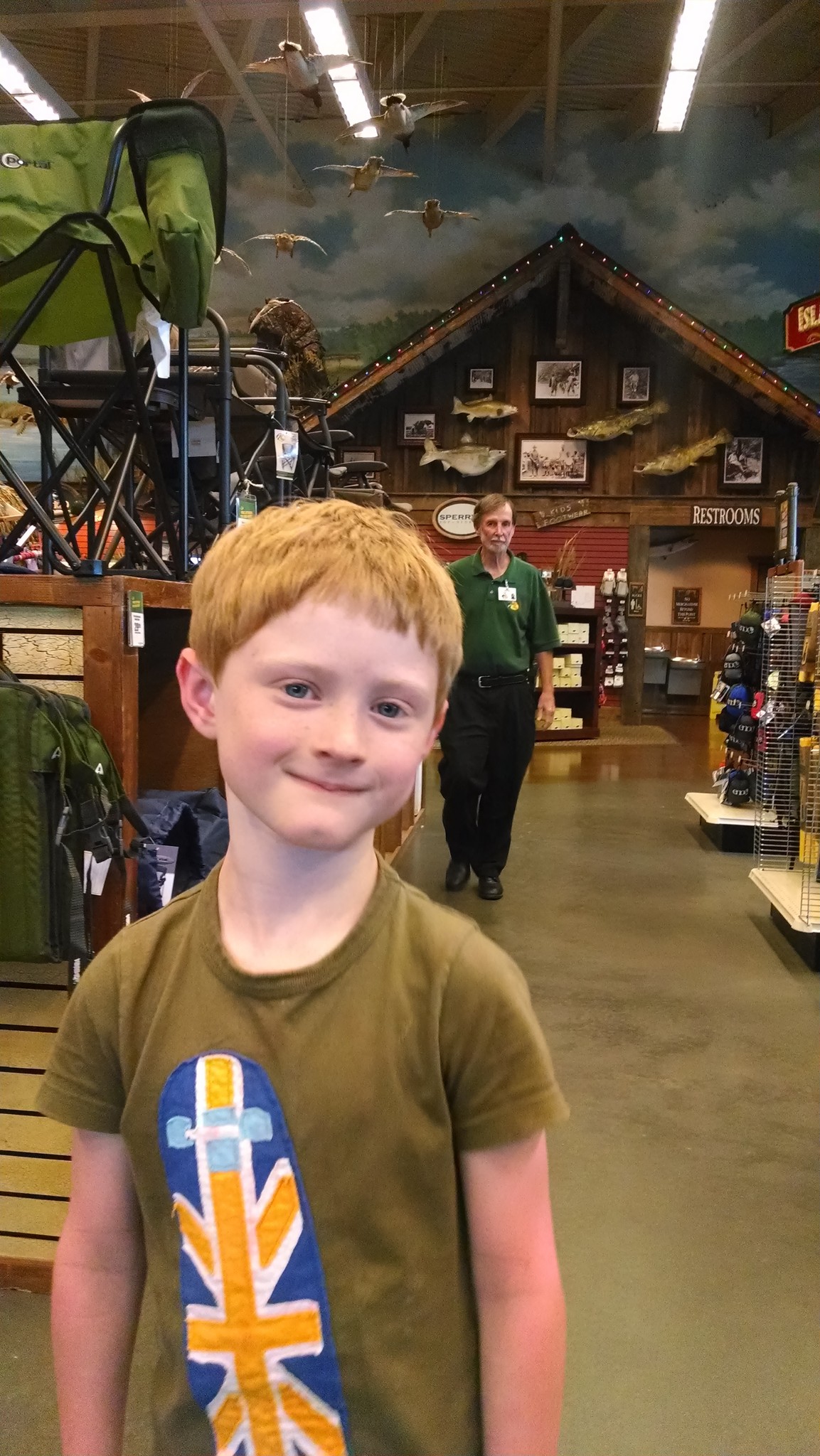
[533, 607, 605, 742]
[0, 574, 427, 1291]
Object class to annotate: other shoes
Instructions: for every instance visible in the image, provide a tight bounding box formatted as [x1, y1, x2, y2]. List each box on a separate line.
[479, 875, 504, 900]
[445, 857, 470, 892]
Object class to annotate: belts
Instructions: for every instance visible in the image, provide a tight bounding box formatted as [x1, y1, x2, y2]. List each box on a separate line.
[457, 668, 533, 688]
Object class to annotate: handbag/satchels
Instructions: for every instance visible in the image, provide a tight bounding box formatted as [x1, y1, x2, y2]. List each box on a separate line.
[136, 786, 229, 919]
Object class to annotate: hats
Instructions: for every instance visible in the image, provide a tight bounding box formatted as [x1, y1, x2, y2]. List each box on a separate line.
[712, 611, 762, 806]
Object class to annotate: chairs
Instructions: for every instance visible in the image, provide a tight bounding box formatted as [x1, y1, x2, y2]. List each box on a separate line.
[0, 98, 412, 582]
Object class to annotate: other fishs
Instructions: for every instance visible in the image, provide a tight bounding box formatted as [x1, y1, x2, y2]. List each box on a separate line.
[450, 395, 520, 423]
[566, 397, 671, 439]
[420, 432, 507, 478]
[636, 427, 735, 477]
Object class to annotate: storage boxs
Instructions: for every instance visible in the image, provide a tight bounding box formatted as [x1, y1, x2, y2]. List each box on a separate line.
[536, 623, 589, 731]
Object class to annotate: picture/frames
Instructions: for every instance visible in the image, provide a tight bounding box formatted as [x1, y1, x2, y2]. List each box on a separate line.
[514, 433, 592, 489]
[616, 362, 654, 409]
[717, 431, 771, 495]
[467, 367, 495, 391]
[338, 446, 381, 484]
[529, 355, 587, 404]
[397, 404, 442, 446]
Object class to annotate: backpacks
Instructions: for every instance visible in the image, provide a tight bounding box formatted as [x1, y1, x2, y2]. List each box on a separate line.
[0, 658, 150, 963]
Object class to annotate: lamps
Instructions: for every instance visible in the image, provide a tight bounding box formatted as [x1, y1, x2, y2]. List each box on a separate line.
[0, 31, 80, 121]
[652, 0, 721, 135]
[299, 0, 383, 139]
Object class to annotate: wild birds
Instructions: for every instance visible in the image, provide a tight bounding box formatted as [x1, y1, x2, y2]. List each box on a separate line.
[309, 156, 418, 197]
[233, 39, 372, 110]
[214, 247, 252, 276]
[333, 93, 468, 154]
[239, 232, 329, 258]
[384, 199, 481, 237]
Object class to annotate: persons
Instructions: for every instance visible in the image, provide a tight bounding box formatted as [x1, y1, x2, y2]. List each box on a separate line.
[529, 443, 586, 481]
[629, 371, 639, 398]
[350, 457, 356, 462]
[728, 445, 752, 474]
[34, 498, 573, 1456]
[473, 372, 488, 382]
[549, 368, 577, 396]
[438, 492, 557, 903]
[411, 422, 427, 436]
